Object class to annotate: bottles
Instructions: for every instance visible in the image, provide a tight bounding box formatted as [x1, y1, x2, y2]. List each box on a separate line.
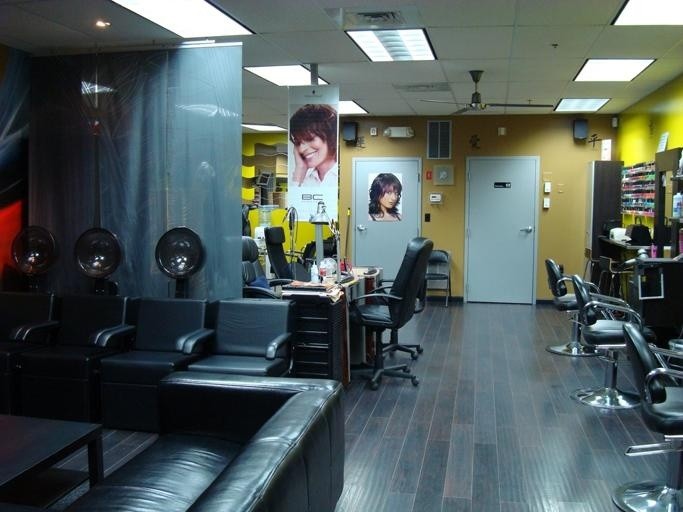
[310, 261, 319, 283]
[672, 193, 682, 218]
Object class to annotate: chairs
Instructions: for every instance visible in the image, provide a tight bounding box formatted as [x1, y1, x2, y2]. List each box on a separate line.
[543, 257, 682, 512]
[241, 226, 293, 301]
[349, 236, 451, 391]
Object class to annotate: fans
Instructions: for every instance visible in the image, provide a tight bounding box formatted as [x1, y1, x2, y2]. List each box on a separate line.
[417, 71, 553, 115]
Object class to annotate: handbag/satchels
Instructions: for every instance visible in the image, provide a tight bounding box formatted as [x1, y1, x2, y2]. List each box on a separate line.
[662, 217, 671, 246]
[625, 215, 651, 246]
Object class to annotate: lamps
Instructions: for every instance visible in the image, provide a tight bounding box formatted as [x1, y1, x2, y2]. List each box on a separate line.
[10, 225, 205, 299]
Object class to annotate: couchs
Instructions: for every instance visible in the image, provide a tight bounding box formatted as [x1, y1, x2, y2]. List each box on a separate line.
[1, 370, 344, 512]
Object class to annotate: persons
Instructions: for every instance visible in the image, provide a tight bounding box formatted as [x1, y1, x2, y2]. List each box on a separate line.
[367, 172, 402, 222]
[288, 104, 338, 224]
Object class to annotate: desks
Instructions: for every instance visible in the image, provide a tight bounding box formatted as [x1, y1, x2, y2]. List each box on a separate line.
[0, 414, 103, 509]
[596, 234, 671, 303]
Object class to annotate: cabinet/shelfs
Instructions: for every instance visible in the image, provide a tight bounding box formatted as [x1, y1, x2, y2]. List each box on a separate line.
[584, 160, 623, 263]
[282, 266, 383, 390]
[629, 258, 682, 339]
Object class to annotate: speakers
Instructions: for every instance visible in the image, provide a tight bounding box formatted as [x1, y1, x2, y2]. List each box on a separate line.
[341, 120, 358, 143]
[573, 117, 589, 140]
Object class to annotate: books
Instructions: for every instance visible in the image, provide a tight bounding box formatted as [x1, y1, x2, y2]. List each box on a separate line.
[281, 280, 341, 298]
